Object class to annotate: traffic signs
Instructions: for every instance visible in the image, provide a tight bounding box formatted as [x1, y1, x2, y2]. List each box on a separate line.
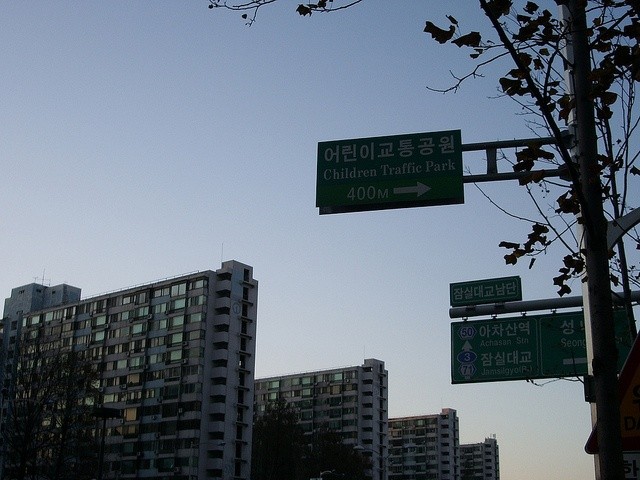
[315, 128, 464, 216]
[449, 275, 637, 384]
